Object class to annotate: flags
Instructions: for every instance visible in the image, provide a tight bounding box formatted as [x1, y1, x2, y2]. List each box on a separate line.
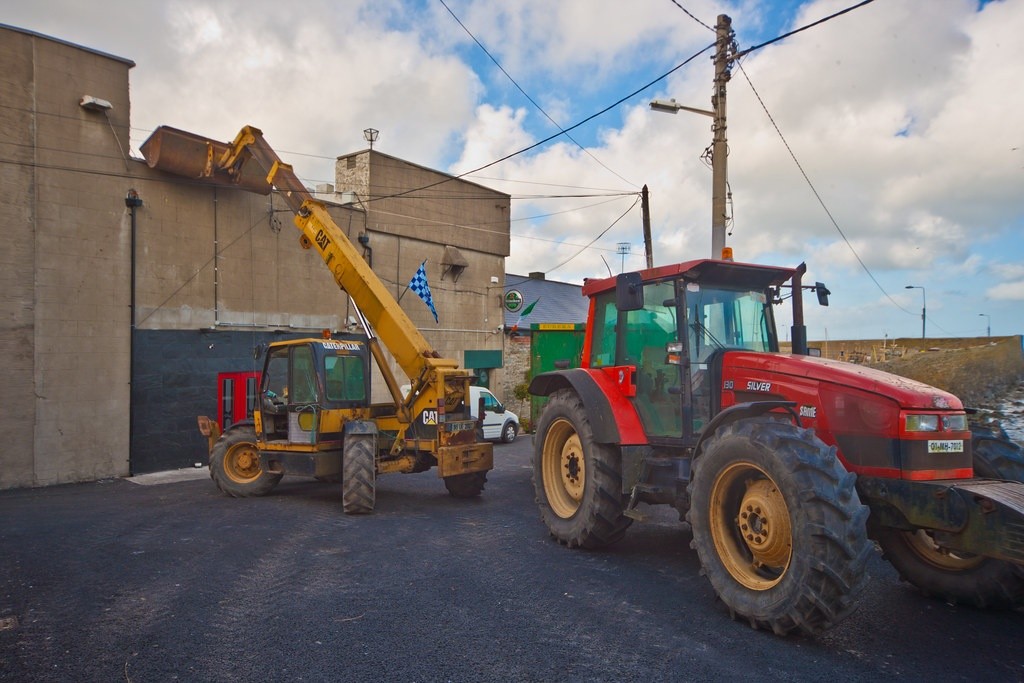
[408, 263, 439, 324]
[520, 297, 540, 320]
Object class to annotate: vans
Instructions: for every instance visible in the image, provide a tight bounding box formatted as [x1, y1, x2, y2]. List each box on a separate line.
[398, 385, 520, 443]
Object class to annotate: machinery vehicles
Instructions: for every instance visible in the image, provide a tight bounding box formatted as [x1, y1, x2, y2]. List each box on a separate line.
[529, 259, 1023, 638]
[140, 125, 496, 515]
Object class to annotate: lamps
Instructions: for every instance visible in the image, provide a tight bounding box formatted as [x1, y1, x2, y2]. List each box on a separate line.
[80, 94, 113, 112]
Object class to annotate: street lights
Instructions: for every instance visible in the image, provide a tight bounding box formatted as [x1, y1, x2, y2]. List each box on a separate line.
[905, 286, 926, 338]
[979, 314, 990, 337]
[649, 101, 726, 261]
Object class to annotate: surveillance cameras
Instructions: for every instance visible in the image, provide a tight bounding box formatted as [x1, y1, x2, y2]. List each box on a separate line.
[497, 323, 504, 331]
[348, 316, 357, 326]
